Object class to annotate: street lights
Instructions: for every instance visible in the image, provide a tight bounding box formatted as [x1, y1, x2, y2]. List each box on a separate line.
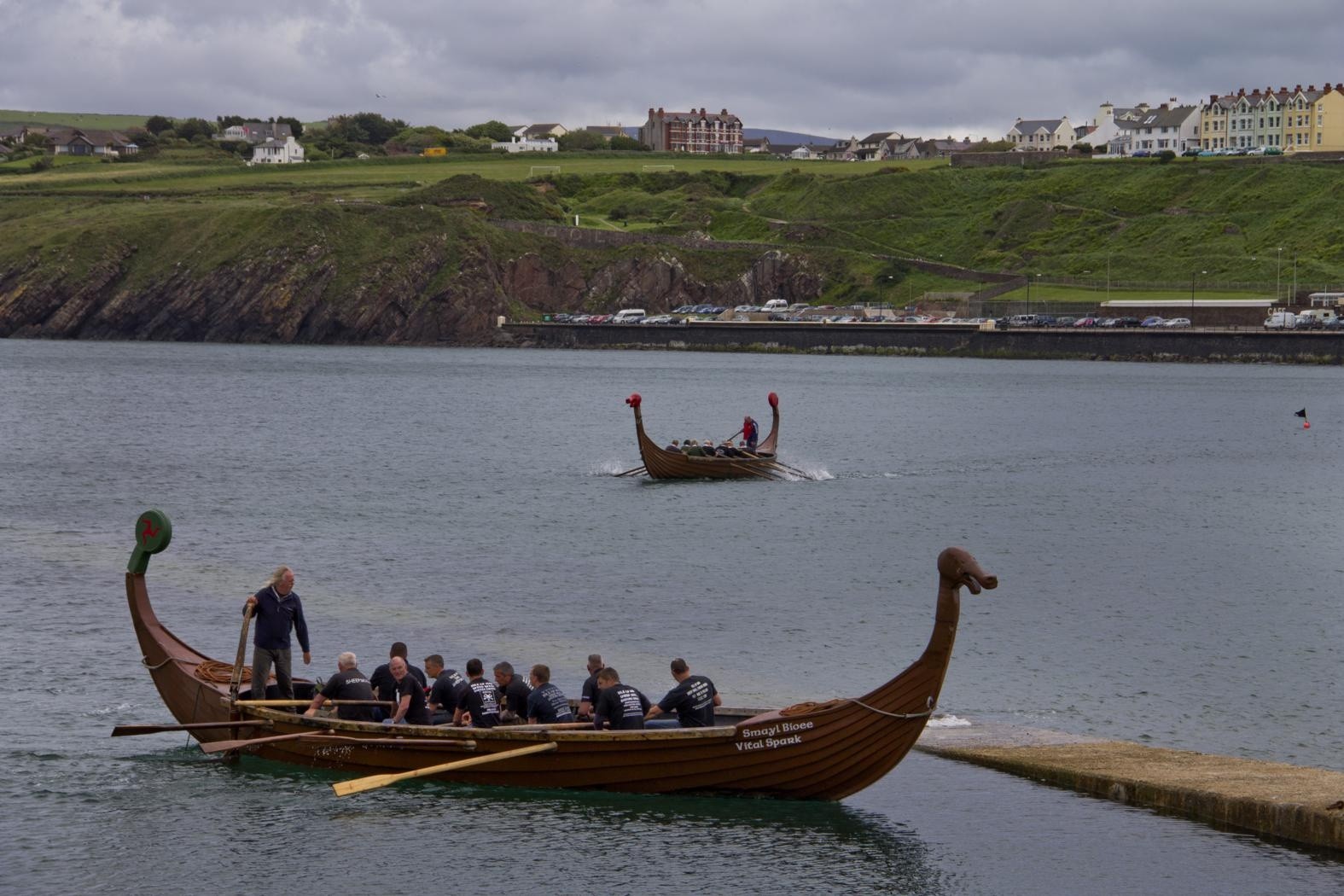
[879, 275, 894, 321]
[1278, 247, 1283, 303]
[1027, 272, 1042, 327]
[1191, 271, 1208, 329]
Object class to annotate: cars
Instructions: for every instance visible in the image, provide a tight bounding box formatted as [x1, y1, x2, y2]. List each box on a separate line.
[670, 304, 733, 314]
[640, 314, 721, 326]
[1166, 317, 1191, 329]
[733, 305, 762, 313]
[541, 312, 615, 324]
[769, 303, 1168, 329]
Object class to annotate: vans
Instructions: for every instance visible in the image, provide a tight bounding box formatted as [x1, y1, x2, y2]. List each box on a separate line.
[612, 308, 646, 324]
[1263, 308, 1344, 332]
[760, 298, 788, 313]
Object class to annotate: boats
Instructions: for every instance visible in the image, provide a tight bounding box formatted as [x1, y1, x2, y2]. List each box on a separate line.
[625, 391, 780, 482]
[124, 508, 1000, 804]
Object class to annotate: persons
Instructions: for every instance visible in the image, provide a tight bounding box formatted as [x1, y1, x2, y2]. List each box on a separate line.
[686, 439, 708, 457]
[666, 439, 683, 454]
[680, 440, 692, 452]
[592, 666, 651, 730]
[702, 438, 755, 458]
[740, 416, 758, 456]
[642, 657, 721, 730]
[241, 564, 311, 714]
[576, 654, 605, 720]
[301, 641, 573, 727]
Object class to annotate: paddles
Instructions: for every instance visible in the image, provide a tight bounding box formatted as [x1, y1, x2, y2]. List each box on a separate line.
[491, 720, 617, 732]
[222, 601, 255, 765]
[641, 473, 650, 477]
[715, 429, 746, 449]
[628, 469, 648, 477]
[613, 465, 646, 478]
[729, 450, 814, 481]
[233, 699, 400, 706]
[198, 729, 329, 755]
[110, 719, 275, 738]
[331, 741, 558, 799]
[298, 734, 410, 749]
